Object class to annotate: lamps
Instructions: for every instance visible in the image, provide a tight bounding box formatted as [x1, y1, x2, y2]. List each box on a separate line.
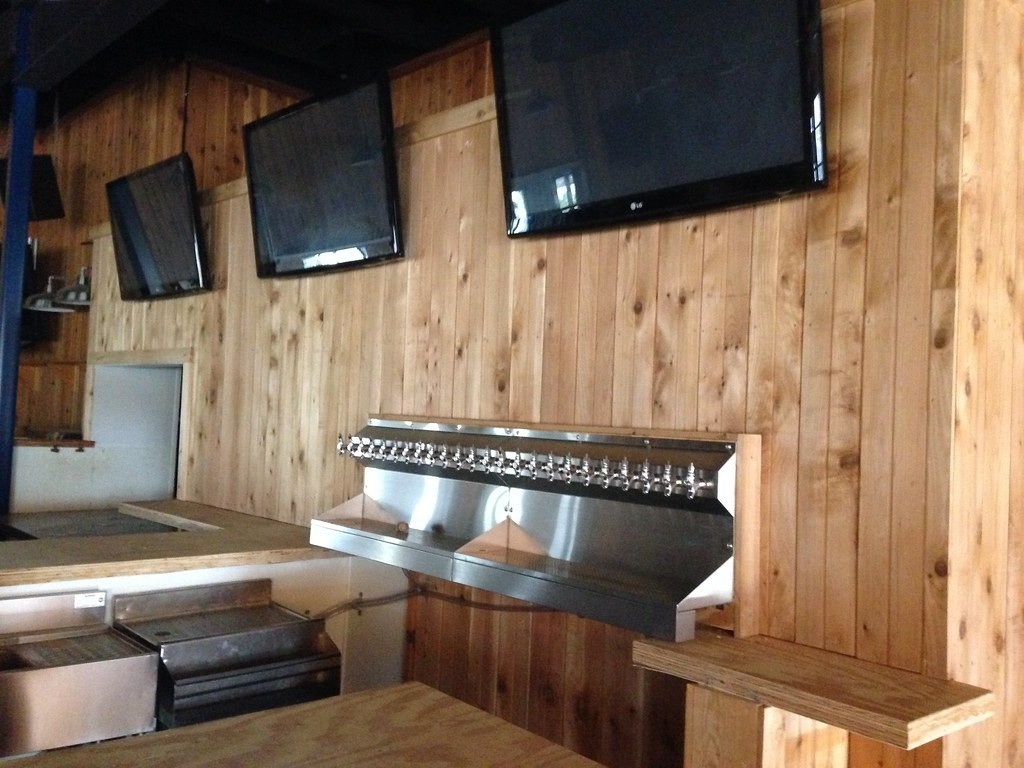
[23, 275, 75, 313]
[54, 266, 90, 306]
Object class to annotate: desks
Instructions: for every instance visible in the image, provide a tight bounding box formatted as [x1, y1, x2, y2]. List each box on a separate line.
[0, 680, 604, 768]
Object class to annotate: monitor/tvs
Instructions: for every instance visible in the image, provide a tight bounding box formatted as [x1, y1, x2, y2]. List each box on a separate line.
[105, 152, 210, 302]
[241, 71, 406, 279]
[491, 0, 829, 238]
